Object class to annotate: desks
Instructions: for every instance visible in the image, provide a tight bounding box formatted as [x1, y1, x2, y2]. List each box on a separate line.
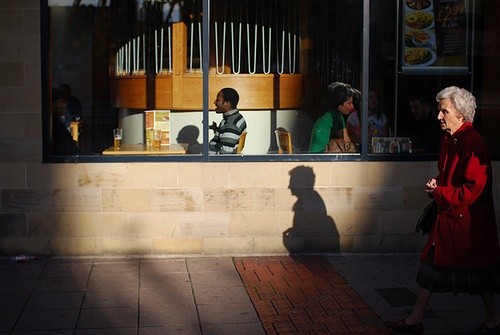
[102, 143, 190, 155]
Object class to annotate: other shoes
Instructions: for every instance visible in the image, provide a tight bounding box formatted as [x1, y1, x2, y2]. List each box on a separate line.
[467, 320, 500, 335]
[384, 318, 424, 334]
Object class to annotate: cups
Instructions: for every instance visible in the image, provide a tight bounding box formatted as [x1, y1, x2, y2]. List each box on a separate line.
[152, 129, 162, 151]
[113, 128, 123, 150]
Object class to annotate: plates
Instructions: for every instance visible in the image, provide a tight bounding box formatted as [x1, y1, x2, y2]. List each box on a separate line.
[403, 11, 434, 29]
[402, 48, 437, 66]
[403, 0, 433, 11]
[403, 29, 436, 48]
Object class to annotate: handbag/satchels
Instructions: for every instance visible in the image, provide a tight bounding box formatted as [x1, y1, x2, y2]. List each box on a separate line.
[415, 200, 437, 236]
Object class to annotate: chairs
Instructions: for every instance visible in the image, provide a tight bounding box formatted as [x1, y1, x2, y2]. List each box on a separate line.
[237, 129, 247, 154]
[274, 129, 292, 154]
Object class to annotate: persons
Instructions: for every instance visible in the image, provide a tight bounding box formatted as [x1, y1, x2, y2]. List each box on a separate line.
[208, 88, 247, 154]
[308, 81, 450, 153]
[381, 86, 500, 335]
[52, 84, 83, 155]
